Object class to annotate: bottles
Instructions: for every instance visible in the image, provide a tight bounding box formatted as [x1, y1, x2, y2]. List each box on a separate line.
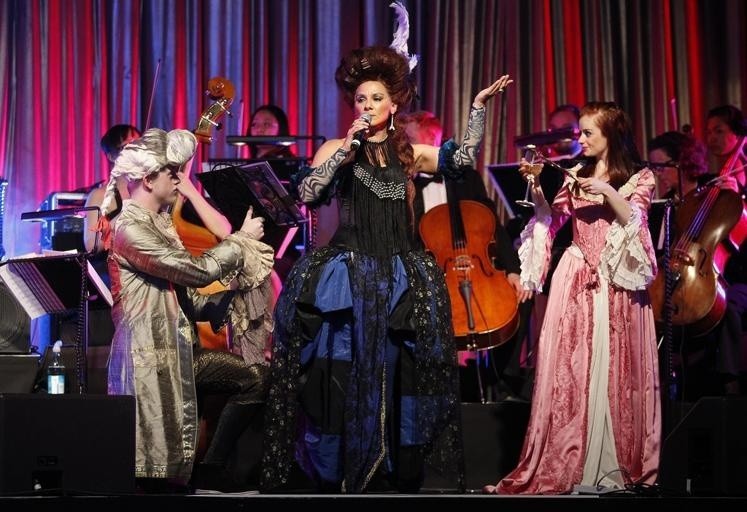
[47, 340, 66, 394]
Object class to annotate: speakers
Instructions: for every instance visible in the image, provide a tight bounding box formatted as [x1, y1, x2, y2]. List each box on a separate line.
[0, 393, 137, 498]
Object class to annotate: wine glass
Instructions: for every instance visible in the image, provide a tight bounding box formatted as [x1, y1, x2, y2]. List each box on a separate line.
[515, 149, 544, 208]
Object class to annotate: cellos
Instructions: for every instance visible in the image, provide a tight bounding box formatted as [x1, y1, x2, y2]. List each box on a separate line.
[647, 138, 747, 334]
[164, 77, 236, 452]
[419, 138, 520, 353]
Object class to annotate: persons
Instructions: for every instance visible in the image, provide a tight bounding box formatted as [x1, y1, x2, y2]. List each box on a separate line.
[644, 131, 746, 394]
[247, 105, 300, 181]
[547, 104, 581, 155]
[260, 46, 514, 495]
[705, 103, 746, 197]
[106, 127, 265, 500]
[482, 102, 658, 493]
[83, 124, 232, 248]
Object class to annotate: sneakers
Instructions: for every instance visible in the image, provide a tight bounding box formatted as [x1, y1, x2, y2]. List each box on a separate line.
[196, 462, 259, 496]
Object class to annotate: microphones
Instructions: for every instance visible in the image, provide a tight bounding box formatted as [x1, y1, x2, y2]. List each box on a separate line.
[349, 113, 372, 150]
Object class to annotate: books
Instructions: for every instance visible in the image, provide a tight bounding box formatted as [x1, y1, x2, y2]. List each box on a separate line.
[236, 161, 309, 226]
[0, 249, 114, 320]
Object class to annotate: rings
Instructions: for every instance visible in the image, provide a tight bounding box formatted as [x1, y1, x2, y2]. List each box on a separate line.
[498, 88, 504, 92]
